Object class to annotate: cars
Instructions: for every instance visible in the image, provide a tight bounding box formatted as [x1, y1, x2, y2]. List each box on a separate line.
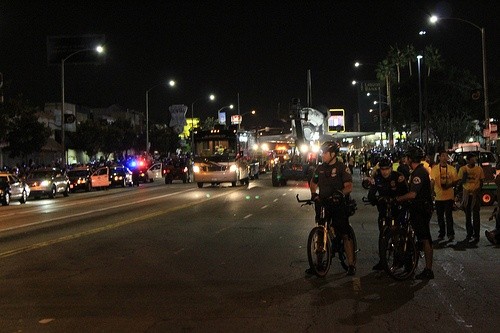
[109, 162, 164, 188]
[0, 171, 30, 206]
[452, 147, 496, 176]
[65, 166, 108, 193]
[24, 167, 72, 198]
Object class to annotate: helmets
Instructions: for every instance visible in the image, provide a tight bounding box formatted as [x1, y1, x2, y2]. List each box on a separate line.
[406, 146, 424, 158]
[378, 157, 393, 167]
[320, 141, 340, 156]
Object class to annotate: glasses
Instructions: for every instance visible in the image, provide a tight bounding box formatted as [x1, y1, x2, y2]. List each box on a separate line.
[380, 167, 390, 171]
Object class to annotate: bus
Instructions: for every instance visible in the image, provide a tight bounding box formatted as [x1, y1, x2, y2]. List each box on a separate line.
[189, 127, 251, 189]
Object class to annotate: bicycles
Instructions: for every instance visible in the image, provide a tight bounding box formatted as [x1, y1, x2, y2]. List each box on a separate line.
[361, 196, 420, 280]
[295, 192, 358, 277]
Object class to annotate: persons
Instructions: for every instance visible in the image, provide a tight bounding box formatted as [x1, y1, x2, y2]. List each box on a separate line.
[0, 140, 500, 283]
[457, 151, 485, 242]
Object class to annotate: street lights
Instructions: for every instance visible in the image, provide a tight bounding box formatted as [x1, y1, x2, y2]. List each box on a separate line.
[145, 77, 176, 157]
[217, 103, 256, 126]
[61, 44, 105, 161]
[430, 14, 489, 143]
[191, 92, 215, 130]
[352, 62, 404, 131]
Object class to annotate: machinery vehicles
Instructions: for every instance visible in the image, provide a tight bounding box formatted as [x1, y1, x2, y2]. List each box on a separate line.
[257, 95, 376, 189]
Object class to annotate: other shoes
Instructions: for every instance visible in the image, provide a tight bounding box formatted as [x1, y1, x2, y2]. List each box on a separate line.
[474, 235, 480, 241]
[448, 235, 455, 241]
[345, 265, 356, 275]
[375, 259, 388, 270]
[415, 268, 434, 279]
[466, 234, 472, 240]
[437, 236, 445, 240]
[306, 263, 322, 273]
